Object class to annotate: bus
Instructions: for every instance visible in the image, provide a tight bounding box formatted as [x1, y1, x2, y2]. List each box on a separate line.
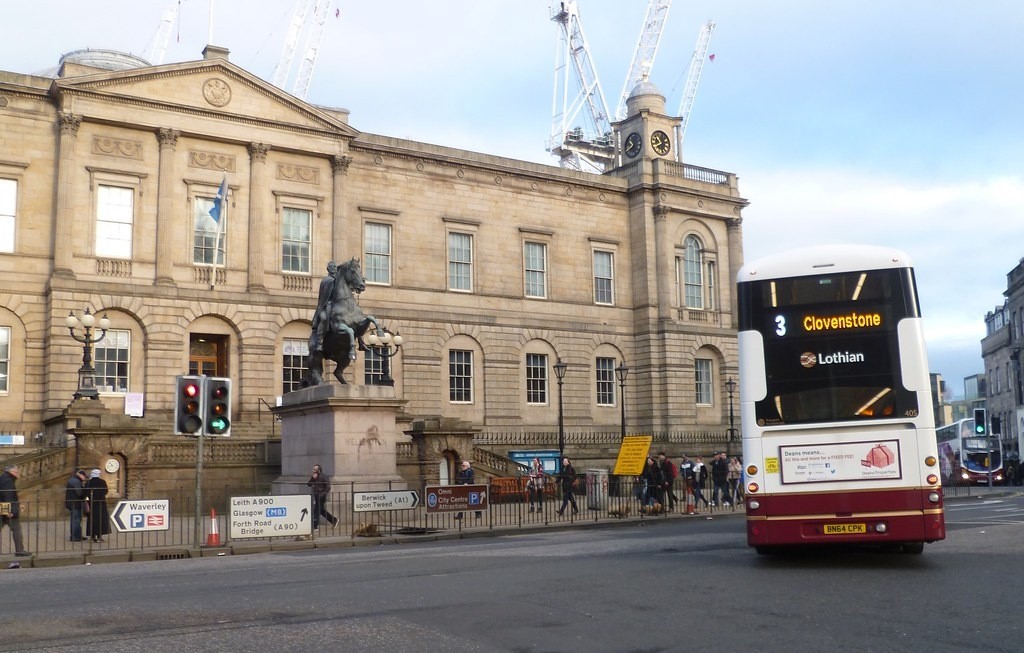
[736, 242, 945, 555]
[936, 418, 1007, 486]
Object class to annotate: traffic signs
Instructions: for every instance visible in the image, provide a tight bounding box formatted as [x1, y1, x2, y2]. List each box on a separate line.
[353, 489, 420, 512]
[424, 484, 489, 514]
[111, 500, 170, 532]
[231, 493, 312, 536]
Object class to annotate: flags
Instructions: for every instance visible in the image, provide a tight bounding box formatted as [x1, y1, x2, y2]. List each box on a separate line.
[209, 176, 229, 224]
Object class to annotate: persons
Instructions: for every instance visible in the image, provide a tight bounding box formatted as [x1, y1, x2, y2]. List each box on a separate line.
[82, 469, 112, 543]
[634, 452, 743, 516]
[65, 470, 89, 542]
[528, 458, 545, 513]
[307, 464, 340, 531]
[312, 262, 370, 351]
[0, 464, 32, 556]
[552, 457, 579, 516]
[454, 461, 482, 519]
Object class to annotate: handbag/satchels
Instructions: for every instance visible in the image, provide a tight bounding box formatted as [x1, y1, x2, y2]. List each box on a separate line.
[83, 501, 90, 514]
[572, 478, 579, 488]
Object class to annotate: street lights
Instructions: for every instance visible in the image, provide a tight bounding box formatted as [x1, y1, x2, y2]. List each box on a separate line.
[725, 376, 737, 443]
[614, 361, 630, 440]
[367, 326, 404, 387]
[553, 356, 568, 473]
[65, 306, 111, 400]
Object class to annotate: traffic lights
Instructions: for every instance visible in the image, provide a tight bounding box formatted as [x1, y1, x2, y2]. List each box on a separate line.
[973, 408, 987, 437]
[204, 377, 232, 437]
[173, 375, 204, 436]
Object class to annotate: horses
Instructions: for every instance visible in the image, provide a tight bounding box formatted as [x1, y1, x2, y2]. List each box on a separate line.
[307, 256, 385, 386]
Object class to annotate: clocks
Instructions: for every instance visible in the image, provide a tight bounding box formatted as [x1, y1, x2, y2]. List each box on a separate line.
[624, 132, 642, 158]
[651, 130, 670, 156]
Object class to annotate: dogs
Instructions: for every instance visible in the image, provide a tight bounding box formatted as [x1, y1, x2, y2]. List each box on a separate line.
[638, 503, 665, 516]
[609, 504, 629, 518]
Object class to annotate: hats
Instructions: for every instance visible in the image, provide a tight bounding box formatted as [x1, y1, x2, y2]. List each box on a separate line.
[78, 471, 89, 478]
[713, 451, 718, 455]
[91, 469, 101, 477]
[657, 452, 666, 456]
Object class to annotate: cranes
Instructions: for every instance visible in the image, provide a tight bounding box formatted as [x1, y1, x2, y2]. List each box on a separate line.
[271, 1, 332, 102]
[142, 0, 182, 66]
[544, 0, 717, 178]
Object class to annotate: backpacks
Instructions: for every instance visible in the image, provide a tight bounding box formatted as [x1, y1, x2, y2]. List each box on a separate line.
[666, 459, 677, 478]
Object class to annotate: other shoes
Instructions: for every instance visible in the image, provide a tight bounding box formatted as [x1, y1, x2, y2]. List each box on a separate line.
[538, 507, 541, 512]
[92, 538, 96, 543]
[454, 516, 462, 519]
[477, 513, 481, 518]
[97, 538, 104, 542]
[82, 537, 87, 540]
[15, 551, 32, 556]
[709, 501, 715, 506]
[723, 501, 730, 506]
[557, 510, 562, 515]
[705, 501, 707, 507]
[573, 508, 578, 514]
[334, 519, 339, 528]
[529, 507, 534, 513]
[314, 528, 319, 531]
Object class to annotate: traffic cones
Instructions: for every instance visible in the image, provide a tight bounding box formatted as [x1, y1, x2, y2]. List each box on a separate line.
[684, 487, 698, 515]
[205, 507, 226, 549]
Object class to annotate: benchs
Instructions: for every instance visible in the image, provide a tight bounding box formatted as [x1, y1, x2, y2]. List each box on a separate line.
[490, 477, 525, 505]
[520, 477, 555, 503]
